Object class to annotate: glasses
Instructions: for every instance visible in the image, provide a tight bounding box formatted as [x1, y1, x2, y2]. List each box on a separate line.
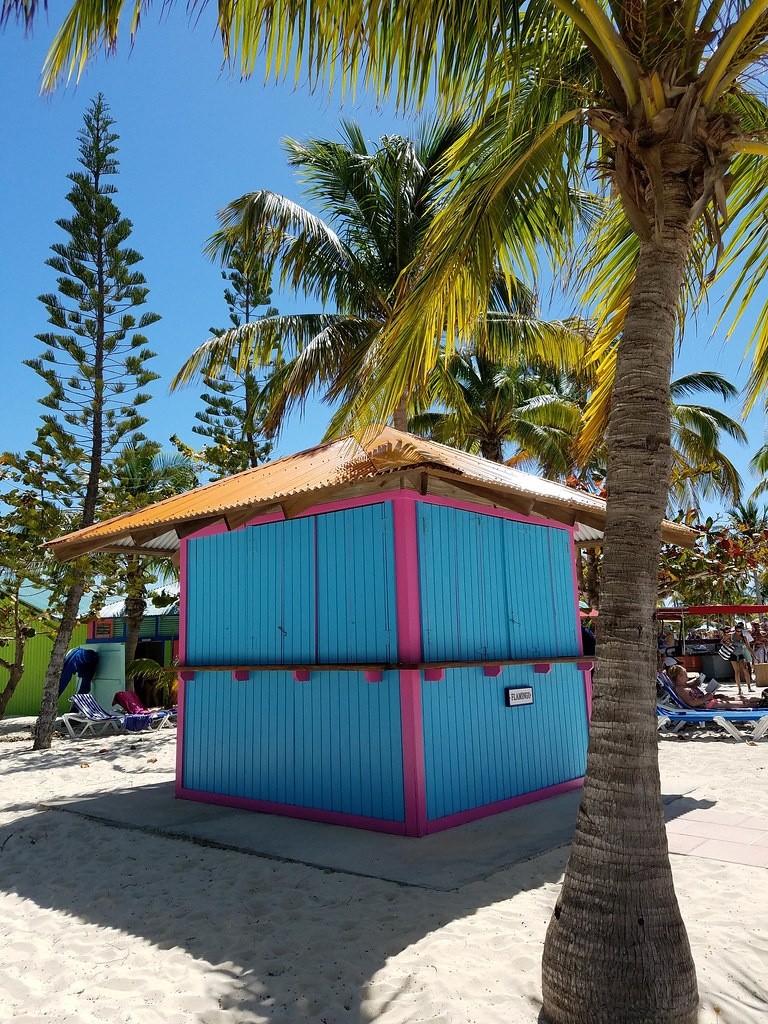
[736, 630, 742, 632]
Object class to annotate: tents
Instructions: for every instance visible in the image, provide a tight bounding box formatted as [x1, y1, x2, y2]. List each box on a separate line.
[657, 605, 768, 657]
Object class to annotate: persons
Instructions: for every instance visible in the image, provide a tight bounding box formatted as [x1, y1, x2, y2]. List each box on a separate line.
[581, 621, 595, 678]
[668, 665, 767, 709]
[721, 624, 761, 695]
[658, 616, 768, 675]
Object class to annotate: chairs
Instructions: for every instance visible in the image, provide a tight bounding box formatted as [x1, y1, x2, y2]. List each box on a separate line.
[656, 704, 768, 742]
[112, 690, 178, 731]
[62, 694, 151, 738]
[656, 672, 768, 734]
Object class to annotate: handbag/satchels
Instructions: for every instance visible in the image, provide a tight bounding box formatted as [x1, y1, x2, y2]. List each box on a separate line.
[718, 635, 735, 661]
